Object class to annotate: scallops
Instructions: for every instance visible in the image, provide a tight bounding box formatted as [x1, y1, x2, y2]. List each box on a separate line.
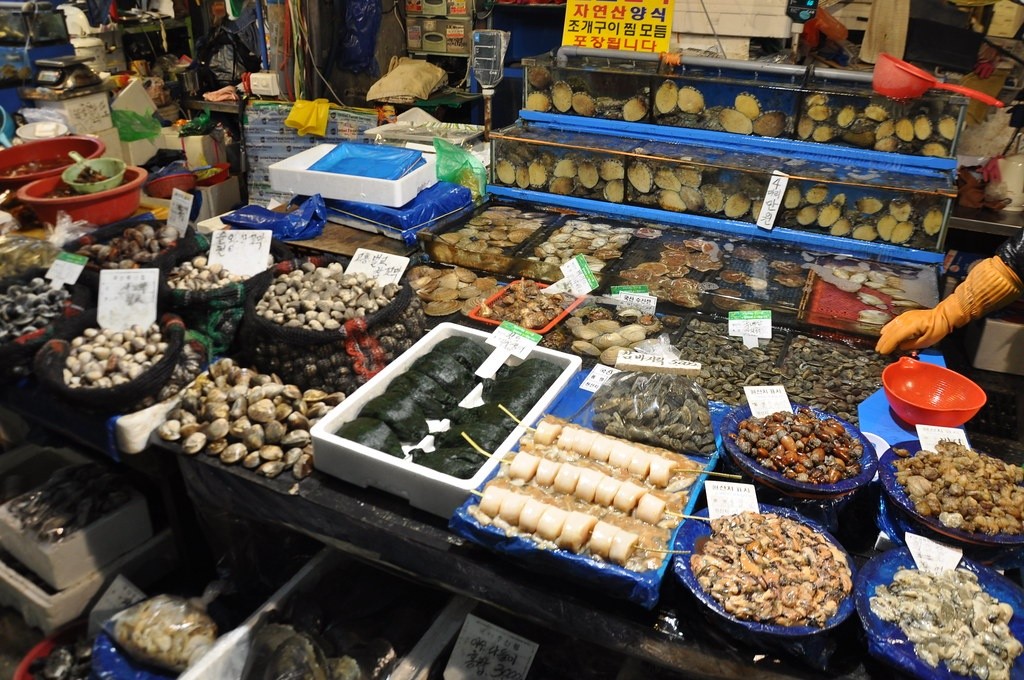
[522, 219, 631, 283]
[620, 227, 802, 311]
[406, 265, 499, 317]
[433, 202, 543, 272]
[566, 307, 664, 366]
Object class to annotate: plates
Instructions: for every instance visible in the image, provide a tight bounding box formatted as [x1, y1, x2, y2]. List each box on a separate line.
[673, 503, 856, 639]
[879, 439, 1023, 547]
[720, 399, 879, 500]
[855, 548, 1024, 680]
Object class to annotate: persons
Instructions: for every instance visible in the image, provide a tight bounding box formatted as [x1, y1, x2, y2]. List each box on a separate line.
[874, 228, 1024, 355]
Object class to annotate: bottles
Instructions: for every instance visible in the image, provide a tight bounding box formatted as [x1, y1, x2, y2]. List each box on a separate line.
[787, 0, 818, 34]
[473, 29, 503, 95]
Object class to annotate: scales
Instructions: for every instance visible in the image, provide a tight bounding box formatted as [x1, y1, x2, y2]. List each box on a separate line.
[35, 55, 97, 88]
[363, 26, 509, 172]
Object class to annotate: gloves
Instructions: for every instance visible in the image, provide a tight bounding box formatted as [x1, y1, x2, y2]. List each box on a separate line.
[875, 255, 1023, 355]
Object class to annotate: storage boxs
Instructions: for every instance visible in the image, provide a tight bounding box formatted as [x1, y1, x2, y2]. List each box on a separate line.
[176, 545, 644, 680]
[26, 94, 240, 227]
[961, 310, 1024, 378]
[447, 368, 726, 612]
[308, 321, 582, 521]
[0, 464, 153, 592]
[245, 104, 441, 208]
[0, 443, 181, 640]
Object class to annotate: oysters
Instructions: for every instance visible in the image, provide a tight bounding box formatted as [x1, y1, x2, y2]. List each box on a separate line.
[782, 334, 889, 421]
[79, 223, 178, 272]
[60, 322, 164, 390]
[0, 278, 70, 339]
[678, 322, 781, 404]
[139, 345, 202, 409]
[167, 257, 228, 292]
[162, 357, 344, 477]
[257, 251, 402, 333]
[198, 298, 426, 394]
[591, 372, 716, 458]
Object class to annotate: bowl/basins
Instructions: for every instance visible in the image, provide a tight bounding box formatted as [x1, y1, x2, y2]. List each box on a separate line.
[0, 103, 230, 227]
[882, 356, 987, 427]
[873, 53, 1004, 109]
